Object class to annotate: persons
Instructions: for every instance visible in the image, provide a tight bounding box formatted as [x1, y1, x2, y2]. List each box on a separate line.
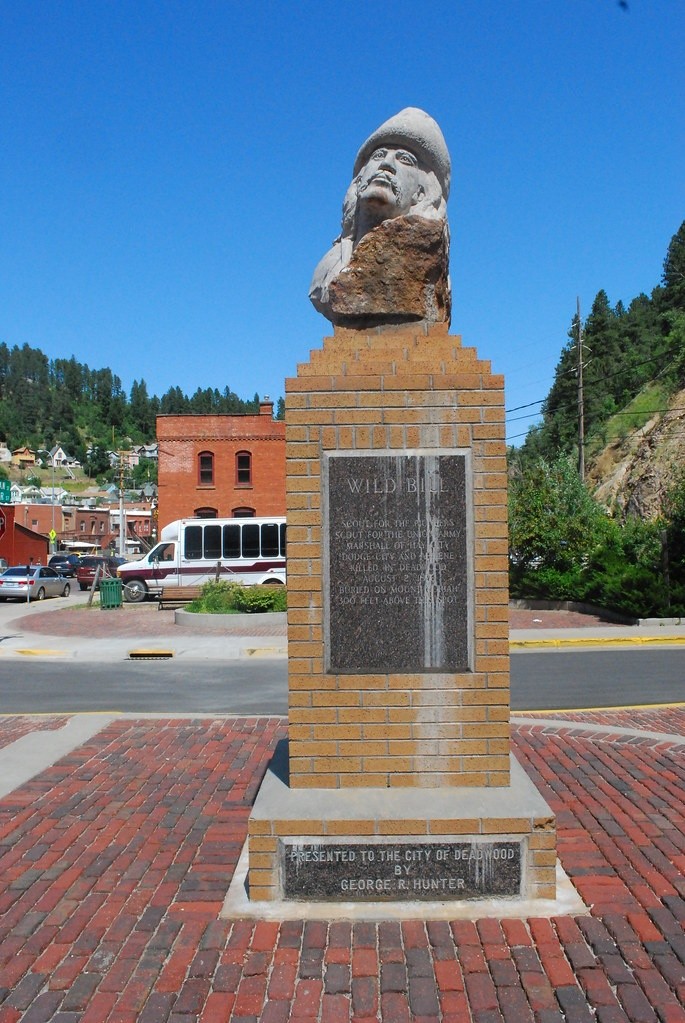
[308, 106, 452, 323]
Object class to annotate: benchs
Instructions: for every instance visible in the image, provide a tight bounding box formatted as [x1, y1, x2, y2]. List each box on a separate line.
[155, 586, 202, 610]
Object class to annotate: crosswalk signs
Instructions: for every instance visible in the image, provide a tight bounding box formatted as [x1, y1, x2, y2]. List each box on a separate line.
[48, 530, 56, 545]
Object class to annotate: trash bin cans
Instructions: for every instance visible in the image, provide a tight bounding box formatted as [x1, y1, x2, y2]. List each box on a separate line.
[99, 578, 123, 610]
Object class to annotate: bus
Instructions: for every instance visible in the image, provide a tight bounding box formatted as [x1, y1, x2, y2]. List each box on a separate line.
[117, 516, 286, 602]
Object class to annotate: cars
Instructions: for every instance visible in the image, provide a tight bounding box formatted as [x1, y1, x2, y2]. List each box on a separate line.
[0, 565, 71, 601]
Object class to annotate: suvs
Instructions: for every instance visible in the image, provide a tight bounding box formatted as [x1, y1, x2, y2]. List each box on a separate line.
[48, 554, 75, 578]
[75, 556, 128, 591]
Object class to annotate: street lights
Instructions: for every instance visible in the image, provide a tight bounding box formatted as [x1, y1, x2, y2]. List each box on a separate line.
[37, 450, 55, 558]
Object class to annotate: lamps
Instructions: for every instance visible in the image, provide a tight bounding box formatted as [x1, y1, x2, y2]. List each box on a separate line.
[131, 445, 175, 457]
